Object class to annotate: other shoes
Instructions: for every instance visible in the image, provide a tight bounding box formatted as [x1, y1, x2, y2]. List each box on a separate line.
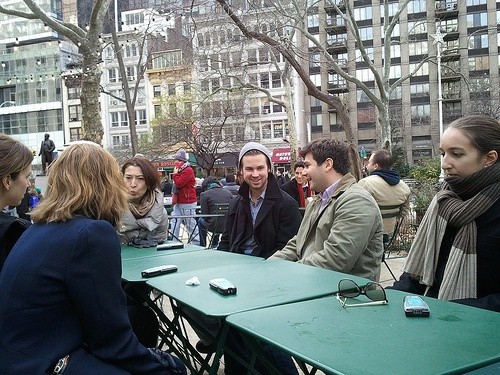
[196, 341, 217, 353]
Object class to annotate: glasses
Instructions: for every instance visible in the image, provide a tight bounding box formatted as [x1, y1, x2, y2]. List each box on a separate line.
[336, 278, 389, 308]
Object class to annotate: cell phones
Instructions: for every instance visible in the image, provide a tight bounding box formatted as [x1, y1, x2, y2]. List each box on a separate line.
[209, 279, 236, 295]
[156, 243, 184, 250]
[403, 295, 430, 317]
[141, 265, 178, 279]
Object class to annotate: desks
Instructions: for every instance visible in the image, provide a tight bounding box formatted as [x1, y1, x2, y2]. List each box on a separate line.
[167, 213, 224, 248]
[146, 259, 373, 375]
[226, 288, 500, 375]
[121, 240, 264, 375]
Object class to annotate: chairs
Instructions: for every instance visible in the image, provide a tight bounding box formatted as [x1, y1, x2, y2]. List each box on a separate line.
[377, 203, 404, 283]
[202, 204, 230, 249]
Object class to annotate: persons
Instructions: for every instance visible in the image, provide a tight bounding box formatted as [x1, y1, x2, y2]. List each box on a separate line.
[0, 136, 43, 272]
[117, 158, 169, 248]
[0, 140, 188, 375]
[160, 169, 291, 197]
[266, 137, 383, 284]
[385, 115, 500, 313]
[358, 149, 411, 243]
[198, 177, 234, 247]
[36, 134, 55, 176]
[171, 148, 200, 245]
[282, 161, 320, 206]
[217, 142, 302, 260]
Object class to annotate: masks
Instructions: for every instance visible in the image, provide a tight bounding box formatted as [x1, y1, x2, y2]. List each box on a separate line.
[174, 162, 184, 168]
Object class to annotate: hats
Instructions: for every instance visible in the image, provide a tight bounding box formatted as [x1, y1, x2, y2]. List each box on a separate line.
[174, 148, 189, 161]
[237, 142, 273, 171]
